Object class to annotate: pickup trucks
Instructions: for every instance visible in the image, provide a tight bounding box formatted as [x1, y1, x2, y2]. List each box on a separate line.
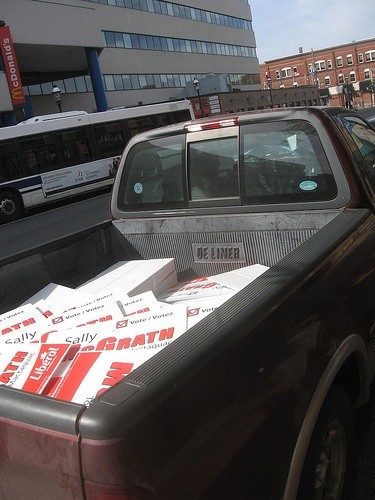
[0, 102, 375, 500]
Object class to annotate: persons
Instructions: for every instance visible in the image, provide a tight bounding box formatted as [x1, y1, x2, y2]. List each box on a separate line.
[197, 152, 231, 191]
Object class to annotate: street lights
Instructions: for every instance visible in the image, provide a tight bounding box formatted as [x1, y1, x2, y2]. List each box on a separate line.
[192, 77, 205, 116]
[51, 84, 62, 113]
[267, 76, 274, 108]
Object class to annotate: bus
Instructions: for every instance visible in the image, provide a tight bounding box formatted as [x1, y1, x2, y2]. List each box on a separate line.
[1, 99, 197, 223]
